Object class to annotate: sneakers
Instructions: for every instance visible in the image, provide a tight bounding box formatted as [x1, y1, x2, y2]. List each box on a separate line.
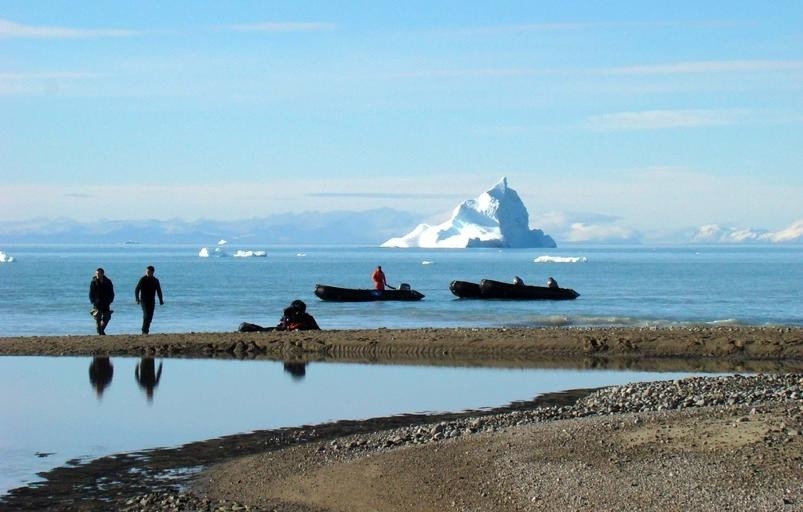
[96, 326, 106, 336]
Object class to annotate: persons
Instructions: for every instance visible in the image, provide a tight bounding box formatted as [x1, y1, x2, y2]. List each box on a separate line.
[371, 266, 386, 290]
[133, 358, 163, 402]
[87, 357, 114, 400]
[87, 266, 115, 336]
[133, 265, 165, 336]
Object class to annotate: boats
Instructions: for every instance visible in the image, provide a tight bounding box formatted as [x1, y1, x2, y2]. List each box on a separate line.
[313, 280, 427, 304]
[446, 273, 581, 306]
